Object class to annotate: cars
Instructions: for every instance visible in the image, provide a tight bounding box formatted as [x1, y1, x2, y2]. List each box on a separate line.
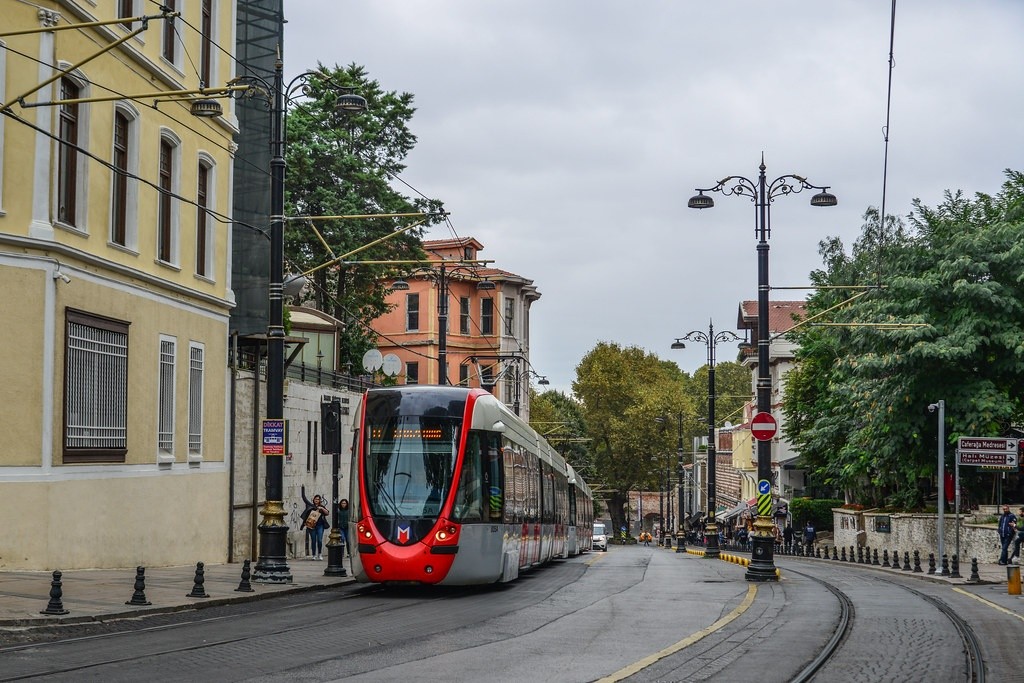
[592, 523, 610, 552]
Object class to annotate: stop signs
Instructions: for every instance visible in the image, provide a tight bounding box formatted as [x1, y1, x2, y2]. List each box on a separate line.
[751, 412, 779, 441]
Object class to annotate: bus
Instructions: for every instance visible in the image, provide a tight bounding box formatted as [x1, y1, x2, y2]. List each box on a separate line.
[346, 386, 596, 588]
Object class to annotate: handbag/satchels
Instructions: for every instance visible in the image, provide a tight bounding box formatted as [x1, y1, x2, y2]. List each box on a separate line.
[304, 509, 321, 530]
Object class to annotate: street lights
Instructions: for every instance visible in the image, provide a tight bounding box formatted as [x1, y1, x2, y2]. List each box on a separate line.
[670, 317, 752, 560]
[687, 151, 838, 583]
[186, 70, 369, 587]
[495, 364, 549, 418]
[387, 262, 497, 386]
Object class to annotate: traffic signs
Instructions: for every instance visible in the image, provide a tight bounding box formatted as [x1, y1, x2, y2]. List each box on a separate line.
[958, 436, 1018, 467]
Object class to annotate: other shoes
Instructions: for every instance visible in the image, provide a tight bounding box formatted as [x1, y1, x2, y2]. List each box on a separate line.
[318, 554, 323, 560]
[312, 556, 316, 560]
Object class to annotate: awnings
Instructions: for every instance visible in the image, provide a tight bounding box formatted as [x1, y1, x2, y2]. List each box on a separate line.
[702, 504, 748, 523]
[688, 511, 703, 526]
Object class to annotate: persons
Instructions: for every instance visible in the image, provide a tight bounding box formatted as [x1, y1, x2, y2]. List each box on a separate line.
[804, 521, 818, 556]
[696, 531, 705, 544]
[337, 499, 349, 557]
[644, 530, 649, 546]
[300, 484, 330, 561]
[772, 525, 783, 545]
[1009, 507, 1024, 561]
[717, 527, 755, 546]
[997, 504, 1017, 565]
[782, 524, 795, 549]
